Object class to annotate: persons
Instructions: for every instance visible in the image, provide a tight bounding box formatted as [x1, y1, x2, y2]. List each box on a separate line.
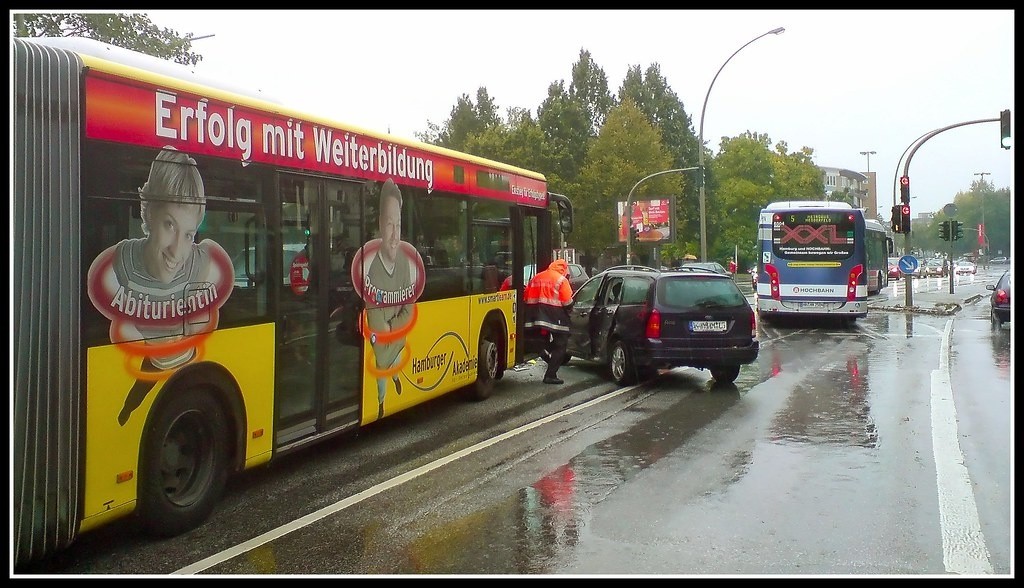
[365, 179, 415, 419]
[729, 259, 737, 279]
[582, 265, 599, 278]
[671, 257, 693, 267]
[892, 245, 949, 280]
[113, 144, 211, 426]
[522, 259, 576, 385]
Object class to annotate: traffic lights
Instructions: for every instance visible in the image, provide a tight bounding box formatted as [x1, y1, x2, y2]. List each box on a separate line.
[698, 165, 706, 188]
[901, 177, 910, 202]
[938, 221, 945, 238]
[891, 207, 899, 233]
[902, 205, 911, 233]
[958, 221, 964, 240]
[304, 228, 310, 236]
[1000, 110, 1011, 151]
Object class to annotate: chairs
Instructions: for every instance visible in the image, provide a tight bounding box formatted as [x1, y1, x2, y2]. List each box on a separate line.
[613, 282, 623, 298]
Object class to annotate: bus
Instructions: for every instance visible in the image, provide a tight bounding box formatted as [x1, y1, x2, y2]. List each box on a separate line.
[866, 218, 894, 293]
[10, 32, 574, 575]
[756, 200, 870, 327]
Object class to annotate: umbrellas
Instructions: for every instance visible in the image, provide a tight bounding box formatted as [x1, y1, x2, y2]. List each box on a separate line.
[684, 255, 697, 263]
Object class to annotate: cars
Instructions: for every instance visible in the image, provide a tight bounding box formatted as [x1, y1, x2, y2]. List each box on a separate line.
[231, 244, 308, 288]
[989, 256, 1007, 264]
[913, 258, 950, 278]
[985, 270, 1011, 330]
[522, 264, 590, 296]
[889, 257, 901, 280]
[956, 261, 977, 275]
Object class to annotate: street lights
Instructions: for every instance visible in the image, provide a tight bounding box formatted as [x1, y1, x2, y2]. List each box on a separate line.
[698, 27, 789, 265]
[859, 151, 876, 173]
[974, 172, 993, 270]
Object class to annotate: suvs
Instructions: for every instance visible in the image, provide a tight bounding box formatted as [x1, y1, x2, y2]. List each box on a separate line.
[564, 265, 759, 387]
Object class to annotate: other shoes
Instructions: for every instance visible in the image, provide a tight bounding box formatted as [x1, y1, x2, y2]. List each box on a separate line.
[543, 374, 565, 384]
